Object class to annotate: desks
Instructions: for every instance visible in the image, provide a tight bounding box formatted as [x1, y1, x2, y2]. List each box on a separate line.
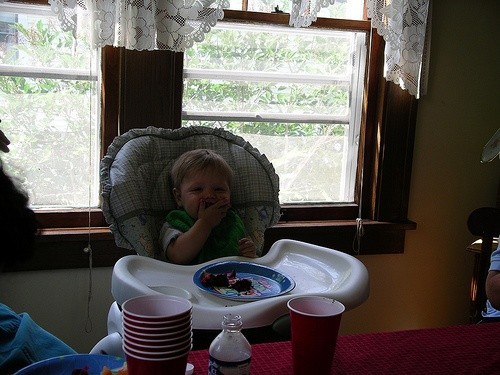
[186, 320, 500, 375]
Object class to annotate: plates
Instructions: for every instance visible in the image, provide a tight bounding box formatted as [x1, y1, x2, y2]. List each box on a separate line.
[193, 260, 296, 302]
[12, 354, 127, 375]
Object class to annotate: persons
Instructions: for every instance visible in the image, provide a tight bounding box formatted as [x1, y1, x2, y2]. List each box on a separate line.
[161, 149, 257, 265]
[475, 235, 499, 325]
[0, 116, 128, 375]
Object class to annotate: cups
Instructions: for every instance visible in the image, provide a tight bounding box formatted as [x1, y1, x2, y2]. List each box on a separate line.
[121, 294, 193, 375]
[287, 296, 345, 375]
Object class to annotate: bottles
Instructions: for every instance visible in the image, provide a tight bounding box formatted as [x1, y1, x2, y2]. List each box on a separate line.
[208, 315, 252, 375]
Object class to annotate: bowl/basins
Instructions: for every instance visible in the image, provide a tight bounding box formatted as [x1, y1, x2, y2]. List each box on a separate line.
[185, 363, 194, 375]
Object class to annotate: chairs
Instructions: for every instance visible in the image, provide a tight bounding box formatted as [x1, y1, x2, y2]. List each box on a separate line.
[98, 126, 283, 262]
